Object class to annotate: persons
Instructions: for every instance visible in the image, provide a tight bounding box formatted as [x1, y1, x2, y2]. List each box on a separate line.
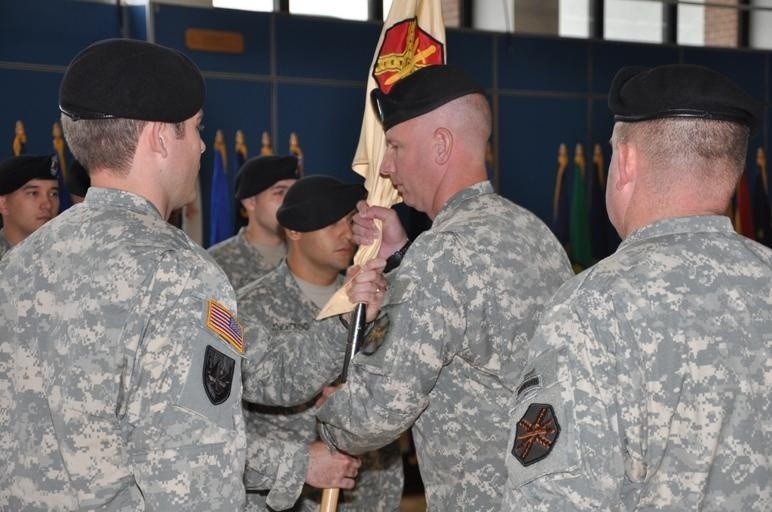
[1, 155, 60, 258]
[316, 65, 574, 511]
[1, 39, 248, 511]
[235, 176, 403, 511]
[504, 64, 771, 511]
[204, 156, 299, 290]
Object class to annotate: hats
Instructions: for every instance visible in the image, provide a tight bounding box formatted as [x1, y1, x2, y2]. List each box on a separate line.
[233, 155, 297, 201]
[58, 40, 206, 123]
[68, 173, 91, 199]
[382, 65, 484, 133]
[276, 175, 366, 233]
[1, 154, 59, 196]
[607, 65, 763, 128]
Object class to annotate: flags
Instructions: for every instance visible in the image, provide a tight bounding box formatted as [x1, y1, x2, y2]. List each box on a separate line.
[314, 0, 446, 510]
[290, 134, 303, 177]
[235, 131, 247, 174]
[734, 145, 771, 247]
[262, 131, 271, 157]
[553, 143, 607, 274]
[209, 128, 236, 248]
[51, 121, 67, 184]
[14, 119, 29, 156]
